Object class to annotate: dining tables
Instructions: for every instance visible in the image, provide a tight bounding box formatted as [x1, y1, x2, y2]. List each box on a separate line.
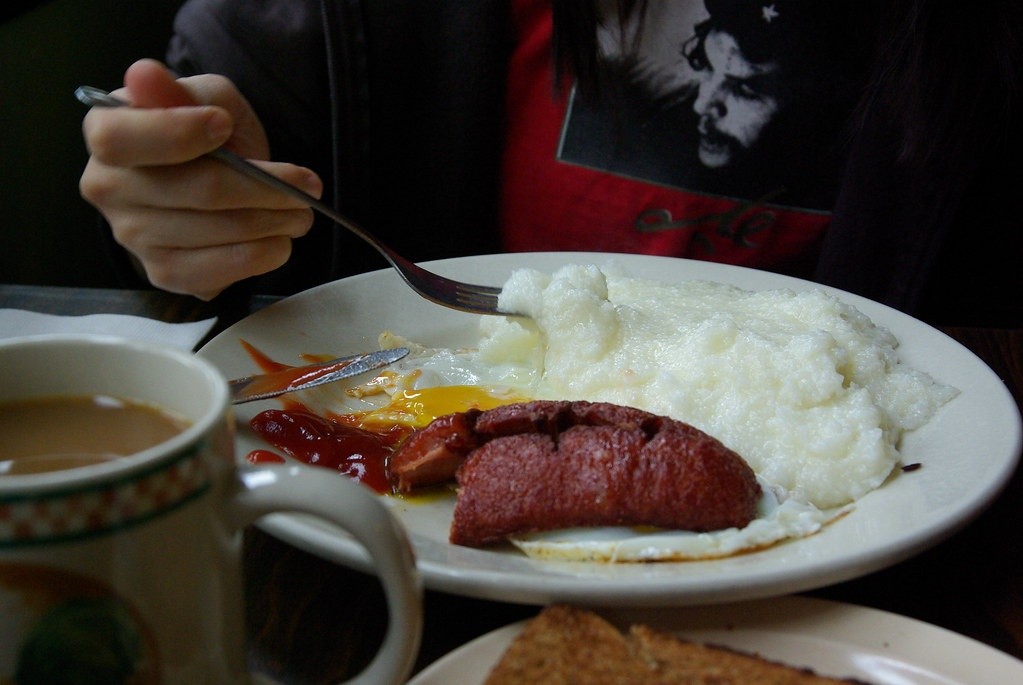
[0, 283, 1023, 685]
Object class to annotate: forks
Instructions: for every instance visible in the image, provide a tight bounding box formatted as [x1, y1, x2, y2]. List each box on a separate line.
[75, 86, 533, 316]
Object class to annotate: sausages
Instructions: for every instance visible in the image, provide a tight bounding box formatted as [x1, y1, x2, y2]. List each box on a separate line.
[387, 400, 766, 547]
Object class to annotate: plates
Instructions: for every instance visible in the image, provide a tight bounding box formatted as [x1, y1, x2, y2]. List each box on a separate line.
[405, 602, 1023, 685]
[192, 252, 1022, 604]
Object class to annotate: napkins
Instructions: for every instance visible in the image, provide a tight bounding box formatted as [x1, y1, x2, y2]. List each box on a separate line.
[0, 307, 218, 352]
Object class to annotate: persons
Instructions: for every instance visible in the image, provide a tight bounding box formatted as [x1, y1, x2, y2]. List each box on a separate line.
[78, 0, 1023, 322]
[677, 0, 797, 171]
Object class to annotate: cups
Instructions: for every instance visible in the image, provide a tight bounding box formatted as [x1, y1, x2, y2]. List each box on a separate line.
[1, 336, 421, 685]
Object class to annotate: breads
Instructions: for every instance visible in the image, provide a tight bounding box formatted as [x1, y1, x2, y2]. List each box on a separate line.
[470, 599, 880, 685]
